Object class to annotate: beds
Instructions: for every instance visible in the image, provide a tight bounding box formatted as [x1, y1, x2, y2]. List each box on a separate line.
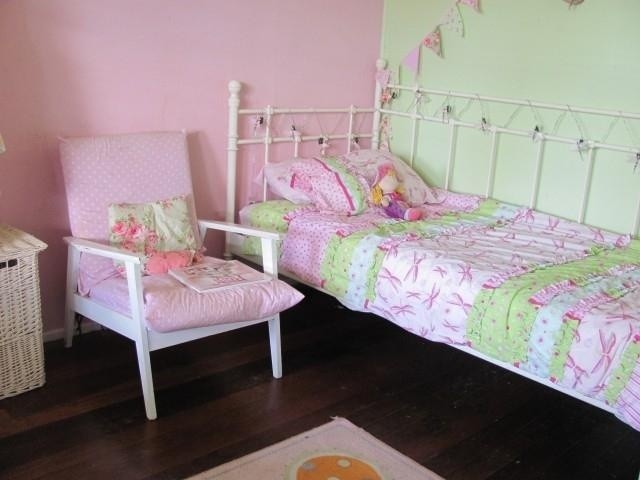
[224, 59, 638, 437]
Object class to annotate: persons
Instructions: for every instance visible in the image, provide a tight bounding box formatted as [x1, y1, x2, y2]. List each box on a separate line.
[372, 162, 423, 221]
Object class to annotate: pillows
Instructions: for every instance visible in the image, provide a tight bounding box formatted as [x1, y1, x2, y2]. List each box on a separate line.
[108, 194, 197, 279]
[263, 147, 444, 215]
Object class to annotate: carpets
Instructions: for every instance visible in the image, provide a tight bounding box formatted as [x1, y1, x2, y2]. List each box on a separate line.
[173, 415, 442, 480]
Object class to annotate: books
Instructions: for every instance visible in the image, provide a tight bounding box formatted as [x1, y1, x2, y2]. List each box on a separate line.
[168, 260, 271, 294]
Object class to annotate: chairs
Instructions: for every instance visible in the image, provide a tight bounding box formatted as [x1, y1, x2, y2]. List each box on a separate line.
[60, 133, 305, 420]
